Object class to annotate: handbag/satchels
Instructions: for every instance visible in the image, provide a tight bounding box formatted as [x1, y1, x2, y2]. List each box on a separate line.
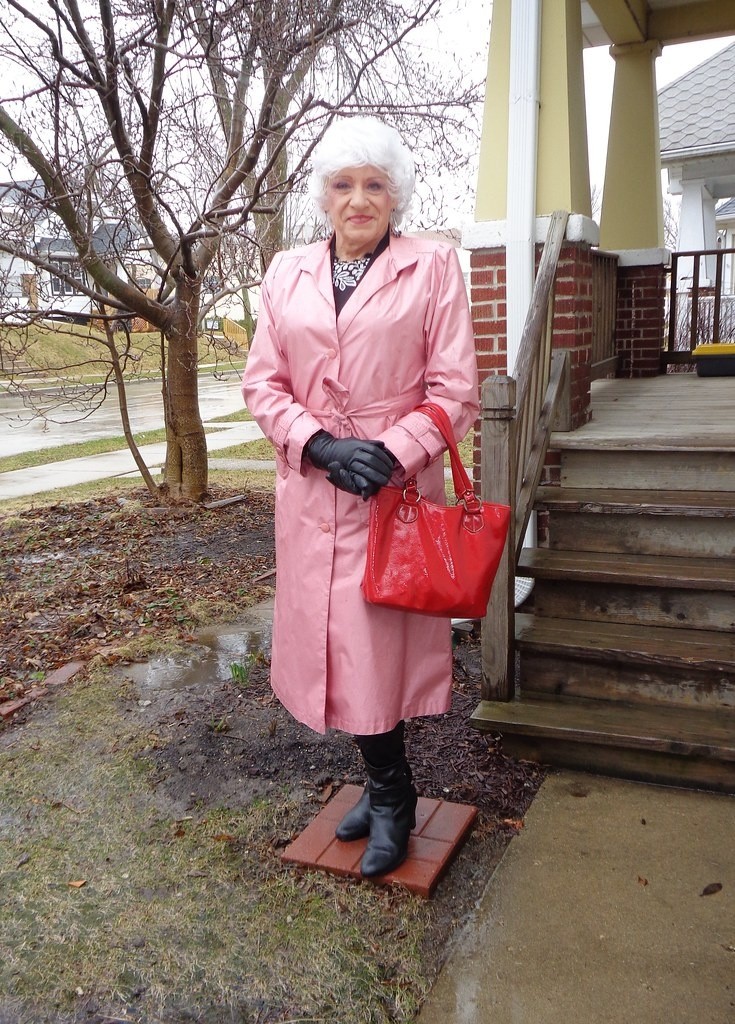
[361, 403, 509, 619]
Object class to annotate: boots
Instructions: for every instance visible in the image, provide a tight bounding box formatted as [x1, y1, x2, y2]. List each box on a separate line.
[361, 755, 418, 877]
[334, 782, 373, 841]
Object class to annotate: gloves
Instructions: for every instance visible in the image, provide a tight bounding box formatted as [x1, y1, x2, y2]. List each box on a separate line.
[307, 432, 395, 489]
[325, 445, 398, 501]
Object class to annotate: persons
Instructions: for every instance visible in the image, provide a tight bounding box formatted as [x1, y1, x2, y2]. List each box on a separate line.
[242, 111, 481, 879]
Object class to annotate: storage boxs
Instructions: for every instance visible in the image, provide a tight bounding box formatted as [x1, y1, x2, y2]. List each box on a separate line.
[693, 343, 735, 377]
